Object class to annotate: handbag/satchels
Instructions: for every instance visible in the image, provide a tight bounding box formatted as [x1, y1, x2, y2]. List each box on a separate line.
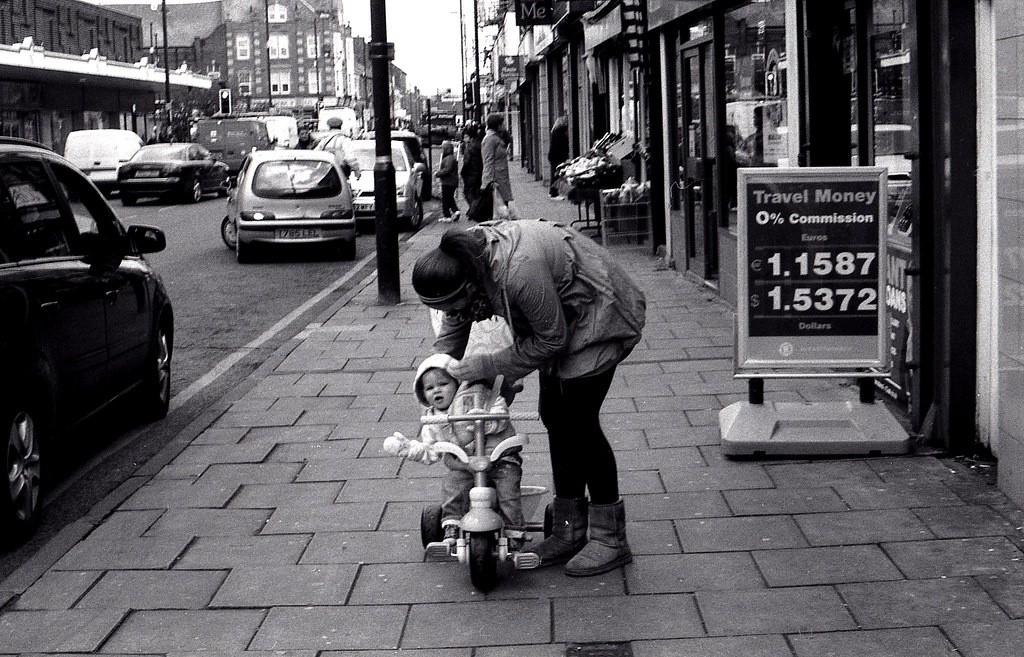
[466, 181, 511, 222]
[460, 316, 513, 362]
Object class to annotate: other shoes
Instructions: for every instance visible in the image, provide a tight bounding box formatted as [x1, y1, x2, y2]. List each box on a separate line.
[442, 537, 457, 554]
[547, 193, 565, 201]
[507, 536, 525, 554]
[453, 211, 461, 222]
[438, 217, 452, 222]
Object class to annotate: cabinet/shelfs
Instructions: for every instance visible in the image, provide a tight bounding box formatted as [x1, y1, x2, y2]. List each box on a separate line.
[599, 189, 653, 256]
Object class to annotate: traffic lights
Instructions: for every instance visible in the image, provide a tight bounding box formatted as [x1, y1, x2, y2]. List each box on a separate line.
[218, 89, 231, 115]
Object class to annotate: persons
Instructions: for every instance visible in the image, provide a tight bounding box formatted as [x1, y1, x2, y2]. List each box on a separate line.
[382, 353, 527, 551]
[411, 218, 647, 576]
[434, 115, 519, 222]
[293, 116, 364, 236]
[152, 121, 191, 144]
[725, 107, 777, 225]
[547, 113, 569, 200]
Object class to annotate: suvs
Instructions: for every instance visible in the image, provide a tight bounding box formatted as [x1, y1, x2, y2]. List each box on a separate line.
[368, 130, 432, 202]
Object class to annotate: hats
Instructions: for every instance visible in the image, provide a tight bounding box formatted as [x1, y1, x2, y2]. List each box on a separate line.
[327, 117, 342, 128]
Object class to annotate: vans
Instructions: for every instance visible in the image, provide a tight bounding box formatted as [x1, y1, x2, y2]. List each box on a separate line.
[318, 107, 356, 130]
[195, 120, 278, 176]
[63, 129, 147, 200]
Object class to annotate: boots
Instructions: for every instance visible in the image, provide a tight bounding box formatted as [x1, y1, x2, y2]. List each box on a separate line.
[565, 495, 632, 575]
[520, 496, 589, 567]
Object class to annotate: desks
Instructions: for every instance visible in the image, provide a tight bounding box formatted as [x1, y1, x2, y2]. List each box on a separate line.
[553, 177, 599, 233]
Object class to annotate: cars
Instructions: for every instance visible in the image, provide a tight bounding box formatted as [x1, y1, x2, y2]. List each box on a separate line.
[117, 142, 231, 205]
[342, 140, 426, 232]
[221, 149, 356, 261]
[0, 135, 177, 552]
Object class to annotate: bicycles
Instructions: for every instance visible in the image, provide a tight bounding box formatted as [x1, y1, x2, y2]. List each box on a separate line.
[417, 411, 549, 592]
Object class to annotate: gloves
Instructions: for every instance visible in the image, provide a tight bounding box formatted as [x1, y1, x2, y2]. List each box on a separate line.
[466, 408, 493, 432]
[384, 432, 424, 463]
